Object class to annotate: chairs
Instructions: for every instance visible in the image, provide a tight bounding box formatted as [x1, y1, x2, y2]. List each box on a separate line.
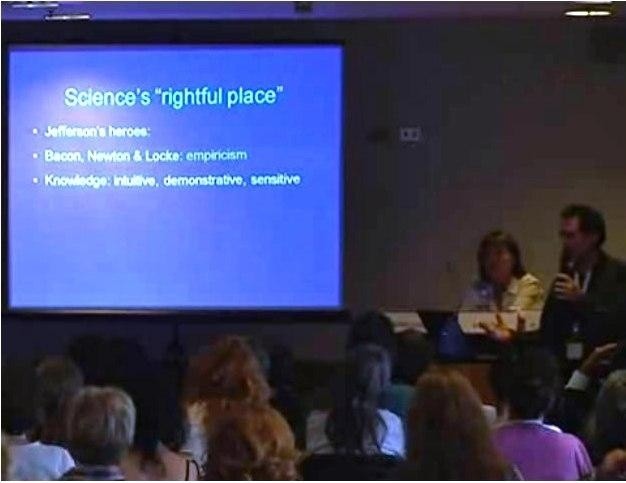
[1, 334, 626, 480]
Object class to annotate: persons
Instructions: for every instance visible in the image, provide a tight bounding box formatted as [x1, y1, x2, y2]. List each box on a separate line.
[1, 309, 625, 480]
[457, 230, 544, 333]
[540, 203, 625, 347]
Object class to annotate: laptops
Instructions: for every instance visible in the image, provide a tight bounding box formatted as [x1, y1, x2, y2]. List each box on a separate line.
[418, 308, 465, 333]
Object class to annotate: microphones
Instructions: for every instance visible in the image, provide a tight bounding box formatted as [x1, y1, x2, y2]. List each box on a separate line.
[557, 258, 575, 280]
